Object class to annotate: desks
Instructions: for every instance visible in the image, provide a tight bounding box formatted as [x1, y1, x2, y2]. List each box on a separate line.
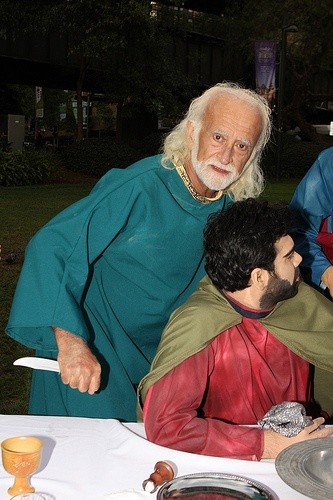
[0, 415, 333, 500]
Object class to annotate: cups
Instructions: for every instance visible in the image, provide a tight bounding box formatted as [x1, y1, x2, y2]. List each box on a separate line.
[9, 492, 56, 500]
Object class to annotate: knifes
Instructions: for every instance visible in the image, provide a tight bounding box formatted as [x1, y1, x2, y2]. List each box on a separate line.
[12, 357, 61, 375]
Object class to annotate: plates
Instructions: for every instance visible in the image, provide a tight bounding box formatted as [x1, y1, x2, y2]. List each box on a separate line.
[156, 472, 280, 500]
[275, 437, 333, 500]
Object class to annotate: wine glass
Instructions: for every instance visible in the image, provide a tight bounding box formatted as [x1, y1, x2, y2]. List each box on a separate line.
[0, 436, 43, 496]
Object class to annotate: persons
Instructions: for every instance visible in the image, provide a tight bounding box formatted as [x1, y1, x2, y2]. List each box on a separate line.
[5, 83, 272, 422]
[288, 121, 333, 303]
[135, 198, 333, 462]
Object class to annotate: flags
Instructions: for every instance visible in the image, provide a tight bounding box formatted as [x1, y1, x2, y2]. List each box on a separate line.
[255, 40, 276, 101]
